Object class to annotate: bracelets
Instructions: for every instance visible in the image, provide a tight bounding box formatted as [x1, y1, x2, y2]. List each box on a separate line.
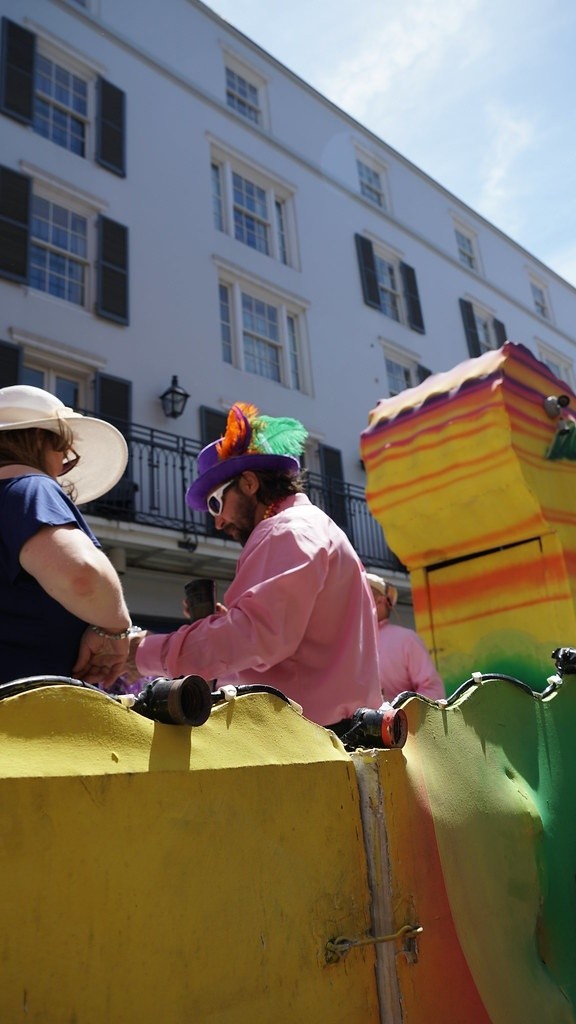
[93, 626, 130, 641]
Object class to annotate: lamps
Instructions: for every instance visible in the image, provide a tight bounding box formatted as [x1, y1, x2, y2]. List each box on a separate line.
[159, 374, 191, 417]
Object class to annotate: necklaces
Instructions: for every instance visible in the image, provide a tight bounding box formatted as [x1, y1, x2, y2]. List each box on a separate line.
[264, 497, 285, 519]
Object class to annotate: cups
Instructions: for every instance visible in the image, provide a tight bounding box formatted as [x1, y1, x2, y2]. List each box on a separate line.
[184, 579, 219, 622]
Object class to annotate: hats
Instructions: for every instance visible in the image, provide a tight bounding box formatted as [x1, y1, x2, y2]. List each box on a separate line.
[187, 402, 309, 512]
[366, 572, 399, 606]
[0, 385, 129, 505]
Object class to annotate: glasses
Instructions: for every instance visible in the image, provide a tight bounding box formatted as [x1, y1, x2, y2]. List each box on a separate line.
[58, 443, 80, 477]
[207, 479, 234, 517]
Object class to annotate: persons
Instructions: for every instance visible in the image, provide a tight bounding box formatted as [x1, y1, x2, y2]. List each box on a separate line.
[365, 572, 446, 707]
[0, 384, 131, 690]
[122, 402, 383, 738]
